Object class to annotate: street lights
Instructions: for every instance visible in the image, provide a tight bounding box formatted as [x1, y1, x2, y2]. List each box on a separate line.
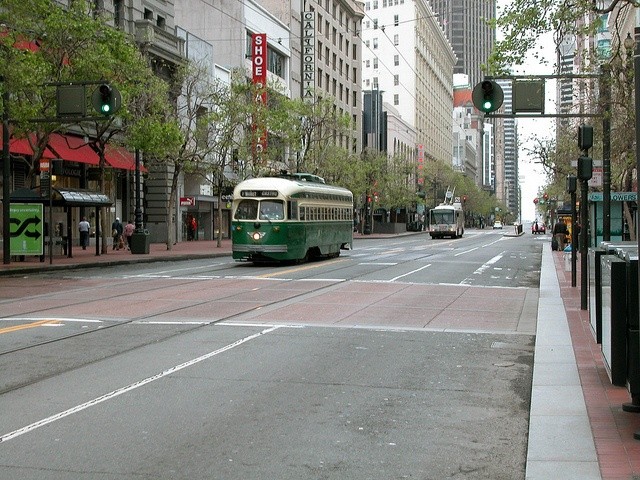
[619, 31, 635, 190]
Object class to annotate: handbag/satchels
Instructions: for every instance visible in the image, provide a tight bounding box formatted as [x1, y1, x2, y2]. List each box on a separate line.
[120, 237, 124, 249]
[112, 229, 117, 237]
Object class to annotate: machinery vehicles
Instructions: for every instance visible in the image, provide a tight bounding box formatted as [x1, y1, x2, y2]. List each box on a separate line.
[428, 185, 465, 239]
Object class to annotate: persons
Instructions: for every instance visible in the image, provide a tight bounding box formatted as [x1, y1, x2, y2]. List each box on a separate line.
[78, 216, 90, 250]
[534, 222, 541, 234]
[111, 217, 123, 250]
[124, 220, 135, 251]
[553, 217, 569, 252]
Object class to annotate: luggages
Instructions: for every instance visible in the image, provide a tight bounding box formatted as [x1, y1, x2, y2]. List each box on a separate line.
[551, 236, 558, 251]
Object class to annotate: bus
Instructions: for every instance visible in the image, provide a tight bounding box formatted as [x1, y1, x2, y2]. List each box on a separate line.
[230, 169, 354, 268]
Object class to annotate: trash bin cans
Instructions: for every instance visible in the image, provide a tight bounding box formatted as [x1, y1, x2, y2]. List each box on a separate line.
[131, 229, 150, 254]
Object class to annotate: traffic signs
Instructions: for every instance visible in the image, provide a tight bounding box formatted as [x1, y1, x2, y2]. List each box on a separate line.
[480, 80, 494, 113]
[97, 84, 113, 116]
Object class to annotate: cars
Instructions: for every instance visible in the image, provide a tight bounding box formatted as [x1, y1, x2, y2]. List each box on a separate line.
[531, 222, 546, 235]
[493, 220, 519, 230]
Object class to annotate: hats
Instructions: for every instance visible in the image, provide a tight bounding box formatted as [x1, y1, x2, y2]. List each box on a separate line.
[115, 218, 120, 221]
